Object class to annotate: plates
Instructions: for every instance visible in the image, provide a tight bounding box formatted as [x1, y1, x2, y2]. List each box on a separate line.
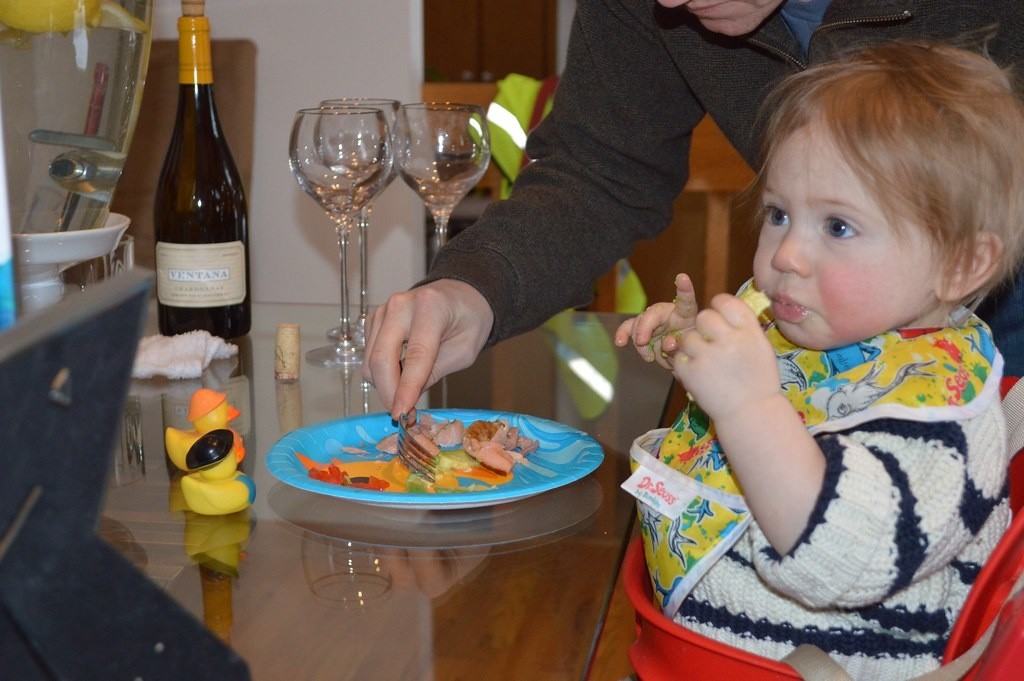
[264, 407, 605, 510]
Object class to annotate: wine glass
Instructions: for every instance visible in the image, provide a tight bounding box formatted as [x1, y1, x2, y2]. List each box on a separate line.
[289, 106, 394, 369]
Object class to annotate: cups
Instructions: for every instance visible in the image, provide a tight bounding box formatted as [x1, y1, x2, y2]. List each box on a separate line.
[320, 97, 412, 342]
[391, 102, 492, 247]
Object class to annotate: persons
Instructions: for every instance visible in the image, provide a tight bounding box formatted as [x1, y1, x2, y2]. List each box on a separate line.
[360, 0, 1024, 426]
[614, 41, 1024, 681]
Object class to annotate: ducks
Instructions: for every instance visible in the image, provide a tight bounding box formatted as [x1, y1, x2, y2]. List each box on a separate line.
[180, 426, 257, 517]
[164, 387, 248, 470]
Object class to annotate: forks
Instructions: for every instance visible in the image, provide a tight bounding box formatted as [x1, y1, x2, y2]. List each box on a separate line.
[399, 341, 439, 483]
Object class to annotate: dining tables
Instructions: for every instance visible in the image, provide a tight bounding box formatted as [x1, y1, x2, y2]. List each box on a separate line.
[0, 301, 674, 681]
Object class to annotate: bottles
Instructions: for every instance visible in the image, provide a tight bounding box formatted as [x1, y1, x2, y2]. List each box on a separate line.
[153, 0, 253, 339]
